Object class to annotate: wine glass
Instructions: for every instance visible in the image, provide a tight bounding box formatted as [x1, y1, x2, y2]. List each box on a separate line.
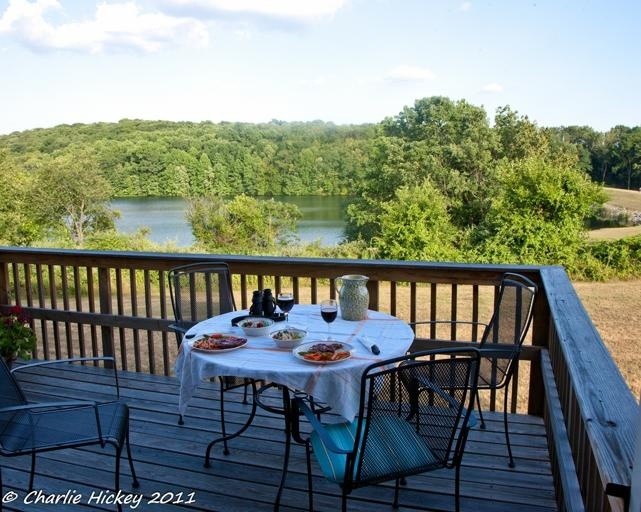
[277, 292, 295, 325]
[320, 299, 339, 342]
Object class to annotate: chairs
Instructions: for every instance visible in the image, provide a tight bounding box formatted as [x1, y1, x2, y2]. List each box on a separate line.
[168, 260, 541, 512]
[0, 354, 140, 512]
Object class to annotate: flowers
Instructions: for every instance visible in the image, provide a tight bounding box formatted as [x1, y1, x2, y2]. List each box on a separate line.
[0, 305, 38, 360]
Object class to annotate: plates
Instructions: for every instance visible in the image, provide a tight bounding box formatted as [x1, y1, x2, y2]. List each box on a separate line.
[187, 332, 249, 354]
[292, 340, 355, 365]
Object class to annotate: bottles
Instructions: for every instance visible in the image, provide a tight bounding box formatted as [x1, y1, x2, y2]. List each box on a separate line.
[249, 289, 277, 319]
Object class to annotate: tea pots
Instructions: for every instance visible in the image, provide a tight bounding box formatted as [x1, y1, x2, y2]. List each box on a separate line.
[333, 275, 370, 322]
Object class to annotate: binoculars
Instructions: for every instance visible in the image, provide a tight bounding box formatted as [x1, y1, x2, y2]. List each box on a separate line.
[249, 289, 276, 317]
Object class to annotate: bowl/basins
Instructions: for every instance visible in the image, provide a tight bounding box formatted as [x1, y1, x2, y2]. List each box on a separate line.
[269, 327, 308, 349]
[238, 317, 275, 337]
